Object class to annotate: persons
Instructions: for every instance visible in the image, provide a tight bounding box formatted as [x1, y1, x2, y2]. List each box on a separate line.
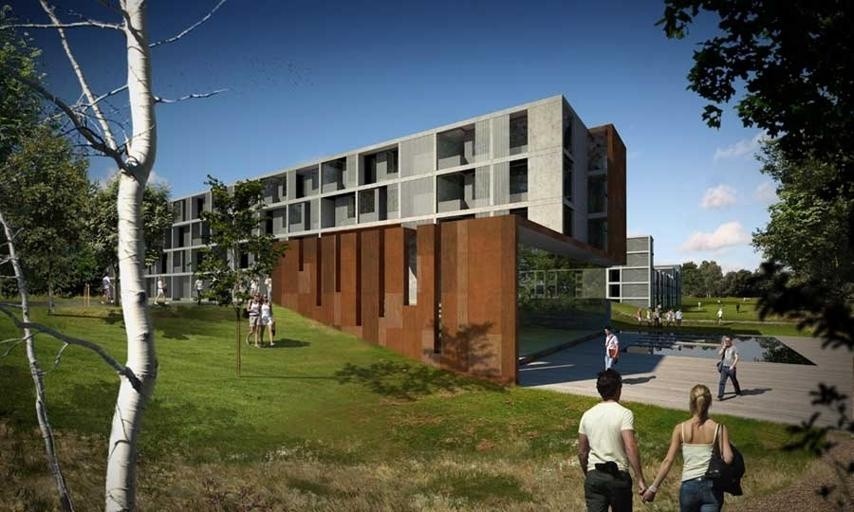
[102, 272, 114, 304]
[735, 302, 740, 316]
[697, 301, 701, 309]
[577, 368, 649, 512]
[194, 271, 276, 349]
[716, 308, 723, 327]
[643, 387, 734, 512]
[717, 298, 722, 306]
[715, 336, 743, 402]
[604, 325, 619, 371]
[153, 275, 169, 304]
[636, 305, 683, 327]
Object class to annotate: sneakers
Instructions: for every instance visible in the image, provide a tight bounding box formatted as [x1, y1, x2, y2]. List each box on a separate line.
[245, 337, 276, 349]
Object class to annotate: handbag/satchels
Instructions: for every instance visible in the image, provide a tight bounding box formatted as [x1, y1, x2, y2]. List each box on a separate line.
[272, 321, 276, 337]
[704, 423, 746, 497]
[242, 299, 252, 320]
[716, 361, 723, 373]
